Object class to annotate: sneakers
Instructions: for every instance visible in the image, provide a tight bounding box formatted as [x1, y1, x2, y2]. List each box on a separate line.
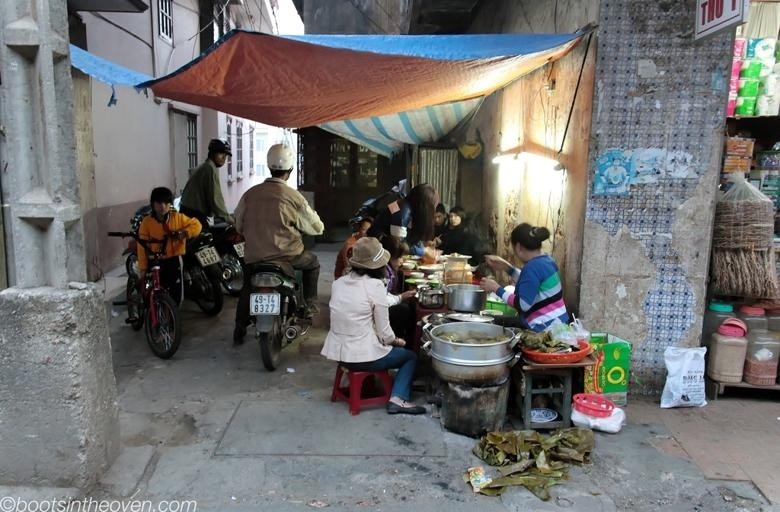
[303, 304, 320, 319]
[386, 401, 426, 414]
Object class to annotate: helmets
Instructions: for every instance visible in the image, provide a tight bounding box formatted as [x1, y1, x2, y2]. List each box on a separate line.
[208, 137, 232, 156]
[267, 144, 294, 170]
[150, 188, 173, 204]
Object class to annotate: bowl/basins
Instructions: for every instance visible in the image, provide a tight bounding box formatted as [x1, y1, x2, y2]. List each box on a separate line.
[419, 290, 445, 309]
[403, 248, 474, 291]
[487, 285, 516, 304]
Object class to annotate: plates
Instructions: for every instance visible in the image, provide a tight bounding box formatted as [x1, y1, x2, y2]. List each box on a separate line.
[719, 317, 748, 338]
[741, 305, 766, 316]
[708, 301, 734, 313]
[529, 408, 558, 423]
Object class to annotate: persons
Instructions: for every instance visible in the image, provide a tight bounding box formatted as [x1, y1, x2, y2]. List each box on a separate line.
[321, 183, 569, 415]
[179, 140, 235, 233]
[137, 187, 203, 334]
[233, 144, 324, 345]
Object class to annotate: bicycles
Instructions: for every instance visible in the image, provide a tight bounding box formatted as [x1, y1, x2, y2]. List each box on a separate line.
[107, 231, 185, 359]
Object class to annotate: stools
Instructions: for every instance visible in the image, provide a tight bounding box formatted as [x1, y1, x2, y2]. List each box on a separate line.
[520, 364, 571, 430]
[331, 364, 391, 415]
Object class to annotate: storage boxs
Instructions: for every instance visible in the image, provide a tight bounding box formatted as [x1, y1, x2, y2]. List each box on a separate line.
[580, 332, 632, 407]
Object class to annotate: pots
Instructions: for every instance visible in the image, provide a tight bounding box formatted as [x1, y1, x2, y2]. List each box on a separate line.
[445, 282, 486, 312]
[421, 321, 524, 360]
[416, 312, 496, 351]
[423, 340, 523, 389]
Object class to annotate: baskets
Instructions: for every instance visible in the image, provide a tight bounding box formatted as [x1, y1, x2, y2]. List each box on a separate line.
[522, 340, 591, 363]
[573, 393, 616, 418]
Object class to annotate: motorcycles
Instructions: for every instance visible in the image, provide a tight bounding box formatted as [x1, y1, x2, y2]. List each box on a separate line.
[211, 222, 246, 299]
[122, 205, 224, 317]
[245, 258, 319, 372]
[346, 188, 392, 229]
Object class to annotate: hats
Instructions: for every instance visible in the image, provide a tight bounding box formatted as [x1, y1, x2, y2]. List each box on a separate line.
[349, 237, 391, 270]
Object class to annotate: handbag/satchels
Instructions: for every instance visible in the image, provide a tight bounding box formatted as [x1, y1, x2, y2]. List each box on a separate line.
[660, 346, 708, 409]
